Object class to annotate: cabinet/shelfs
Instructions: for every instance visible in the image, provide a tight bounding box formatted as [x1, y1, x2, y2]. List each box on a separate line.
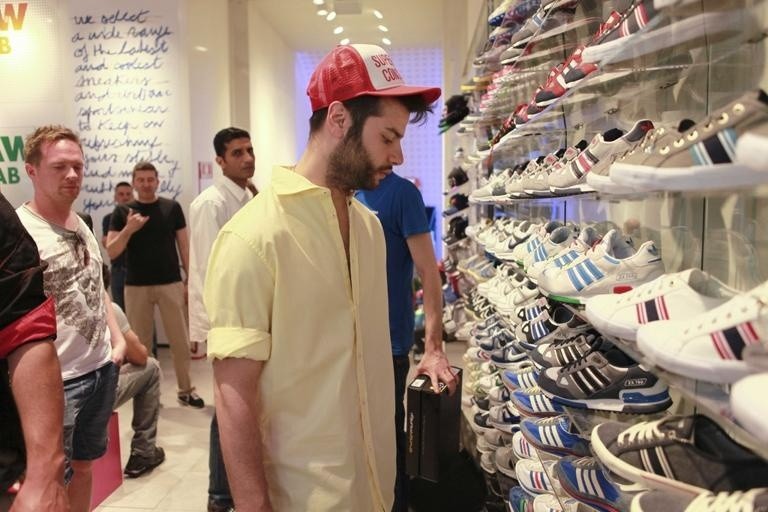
[452, 1, 767, 512]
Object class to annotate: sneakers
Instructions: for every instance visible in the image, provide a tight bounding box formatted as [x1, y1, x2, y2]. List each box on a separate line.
[123, 446, 165, 477]
[461, 0, 768, 512]
[177, 390, 204, 409]
[413, 255, 464, 367]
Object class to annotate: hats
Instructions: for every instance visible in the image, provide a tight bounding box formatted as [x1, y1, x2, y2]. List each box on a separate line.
[439, 92, 470, 245]
[308, 41, 440, 112]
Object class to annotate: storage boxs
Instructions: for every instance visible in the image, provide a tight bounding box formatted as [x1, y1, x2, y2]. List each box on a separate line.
[406, 366, 464, 483]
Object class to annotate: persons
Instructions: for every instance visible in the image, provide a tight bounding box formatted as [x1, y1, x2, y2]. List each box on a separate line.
[104, 159, 208, 409]
[0, 190, 75, 511]
[350, 168, 461, 510]
[200, 43, 444, 511]
[100, 181, 158, 357]
[186, 126, 260, 512]
[101, 261, 166, 477]
[15, 124, 129, 510]
